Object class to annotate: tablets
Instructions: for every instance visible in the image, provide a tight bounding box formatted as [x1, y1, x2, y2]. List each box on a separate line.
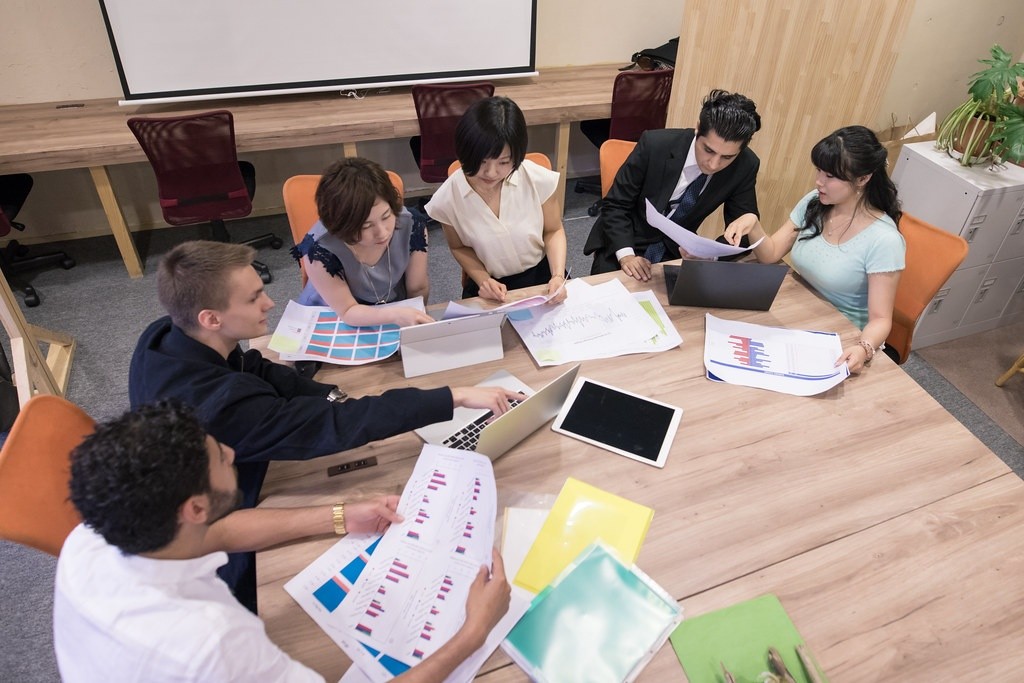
[550, 377, 683, 470]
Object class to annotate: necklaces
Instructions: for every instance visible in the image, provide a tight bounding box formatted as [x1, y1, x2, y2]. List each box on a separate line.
[348, 241, 391, 306]
[827, 206, 867, 236]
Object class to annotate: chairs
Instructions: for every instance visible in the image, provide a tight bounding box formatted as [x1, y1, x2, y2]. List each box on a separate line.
[410, 84, 493, 224]
[283, 169, 403, 289]
[448, 152, 553, 289]
[880, 214, 970, 364]
[573, 69, 674, 216]
[0, 175, 76, 306]
[0, 395, 104, 557]
[125, 110, 284, 282]
[600, 139, 637, 201]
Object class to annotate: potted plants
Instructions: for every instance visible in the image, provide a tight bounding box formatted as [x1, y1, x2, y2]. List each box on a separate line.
[936, 44, 1024, 167]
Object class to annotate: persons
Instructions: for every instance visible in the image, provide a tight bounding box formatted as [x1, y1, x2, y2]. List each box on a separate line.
[584, 89, 761, 275]
[723, 125, 906, 376]
[423, 95, 568, 306]
[123, 241, 529, 612]
[51, 395, 510, 683]
[290, 156, 434, 328]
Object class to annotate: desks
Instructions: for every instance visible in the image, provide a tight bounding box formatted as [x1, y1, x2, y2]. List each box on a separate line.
[0, 61, 672, 277]
[251, 253, 1024, 683]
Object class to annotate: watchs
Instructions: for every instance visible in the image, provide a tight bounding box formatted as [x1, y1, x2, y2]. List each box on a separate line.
[327, 388, 347, 403]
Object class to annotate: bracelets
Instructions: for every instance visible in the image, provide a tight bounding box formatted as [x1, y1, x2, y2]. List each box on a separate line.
[333, 501, 348, 535]
[550, 274, 568, 284]
[855, 340, 874, 363]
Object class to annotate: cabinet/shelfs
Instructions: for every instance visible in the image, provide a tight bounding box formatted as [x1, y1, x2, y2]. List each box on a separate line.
[886, 137, 1024, 351]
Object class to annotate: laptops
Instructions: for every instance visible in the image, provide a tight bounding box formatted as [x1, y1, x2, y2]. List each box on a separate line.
[663, 259, 790, 312]
[414, 363, 583, 461]
[396, 303, 508, 356]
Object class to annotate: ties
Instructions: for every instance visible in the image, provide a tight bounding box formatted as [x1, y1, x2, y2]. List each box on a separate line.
[644, 172, 710, 263]
